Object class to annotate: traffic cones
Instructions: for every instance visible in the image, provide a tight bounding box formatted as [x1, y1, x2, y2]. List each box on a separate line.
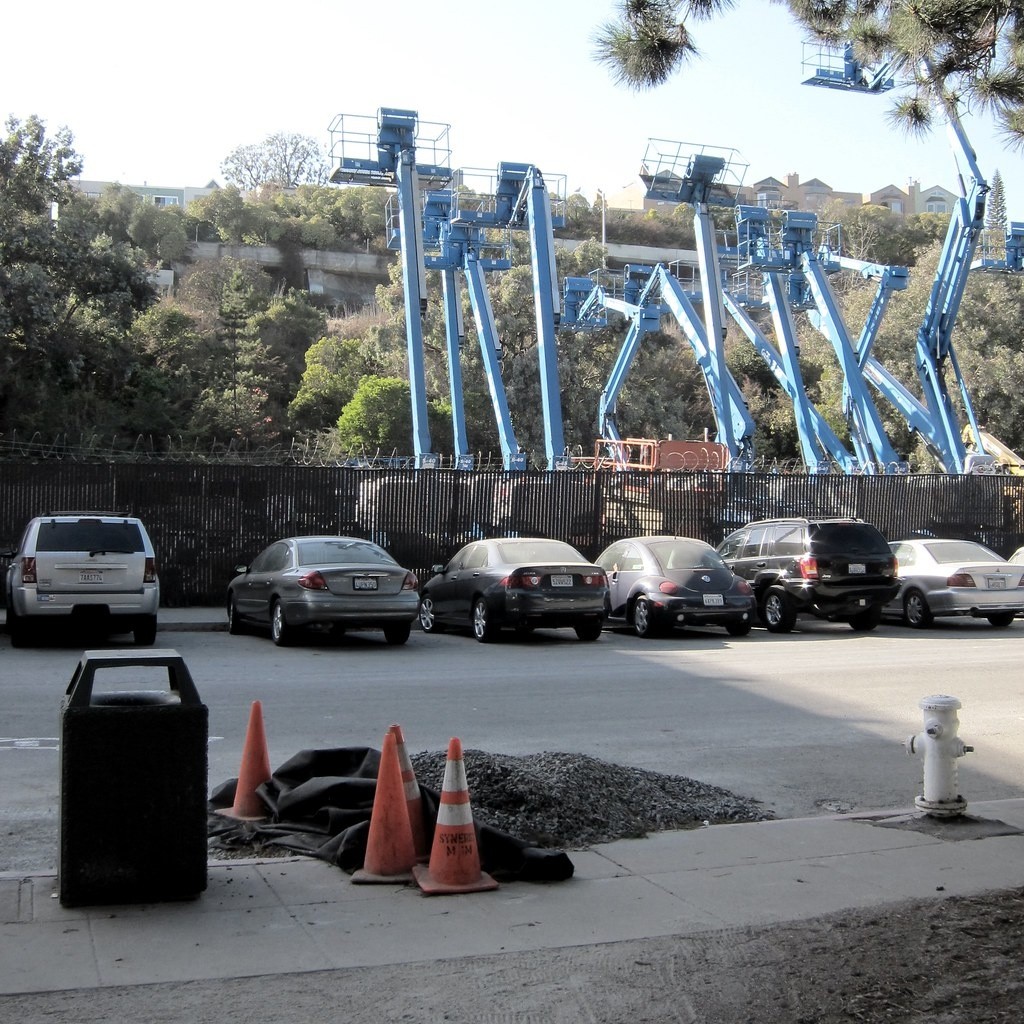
[212, 698, 274, 822]
[411, 736, 500, 892]
[350, 730, 426, 884]
[387, 721, 427, 863]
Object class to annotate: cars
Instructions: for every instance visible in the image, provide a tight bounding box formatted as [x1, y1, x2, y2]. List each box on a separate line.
[593, 535, 759, 640]
[222, 535, 421, 646]
[881, 537, 1024, 629]
[417, 538, 613, 644]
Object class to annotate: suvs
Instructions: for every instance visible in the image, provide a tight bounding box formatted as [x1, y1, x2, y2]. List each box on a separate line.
[0, 503, 161, 649]
[714, 514, 902, 635]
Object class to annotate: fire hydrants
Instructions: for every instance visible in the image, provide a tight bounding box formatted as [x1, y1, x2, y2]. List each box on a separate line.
[901, 693, 975, 818]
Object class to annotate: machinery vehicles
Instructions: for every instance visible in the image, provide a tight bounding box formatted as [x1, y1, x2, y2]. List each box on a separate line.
[323, 26, 1023, 536]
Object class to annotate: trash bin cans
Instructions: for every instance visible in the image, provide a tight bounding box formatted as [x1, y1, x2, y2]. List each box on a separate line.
[57, 649, 209, 909]
